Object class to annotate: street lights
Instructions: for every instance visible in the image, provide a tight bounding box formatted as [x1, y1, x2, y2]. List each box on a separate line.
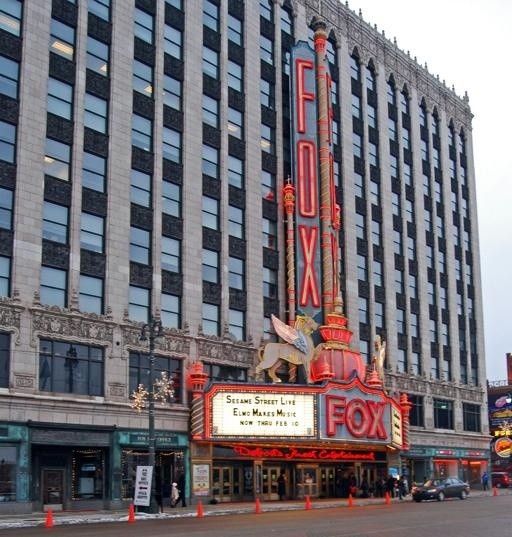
[138, 313, 166, 514]
[65, 346, 78, 395]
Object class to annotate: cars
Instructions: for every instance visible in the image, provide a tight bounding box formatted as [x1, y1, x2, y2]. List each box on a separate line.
[492, 470, 511, 487]
[412, 476, 470, 502]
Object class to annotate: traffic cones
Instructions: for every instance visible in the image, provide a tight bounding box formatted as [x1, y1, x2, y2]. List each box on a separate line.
[45, 507, 54, 529]
[256, 497, 262, 513]
[493, 486, 497, 496]
[198, 500, 203, 518]
[127, 503, 135, 524]
[348, 492, 353, 506]
[385, 490, 391, 504]
[305, 495, 312, 510]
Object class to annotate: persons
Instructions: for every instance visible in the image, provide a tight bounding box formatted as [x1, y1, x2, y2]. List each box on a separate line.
[277, 472, 286, 501]
[170, 482, 181, 508]
[424, 477, 428, 484]
[176, 471, 187, 507]
[482, 472, 489, 491]
[374, 474, 405, 500]
